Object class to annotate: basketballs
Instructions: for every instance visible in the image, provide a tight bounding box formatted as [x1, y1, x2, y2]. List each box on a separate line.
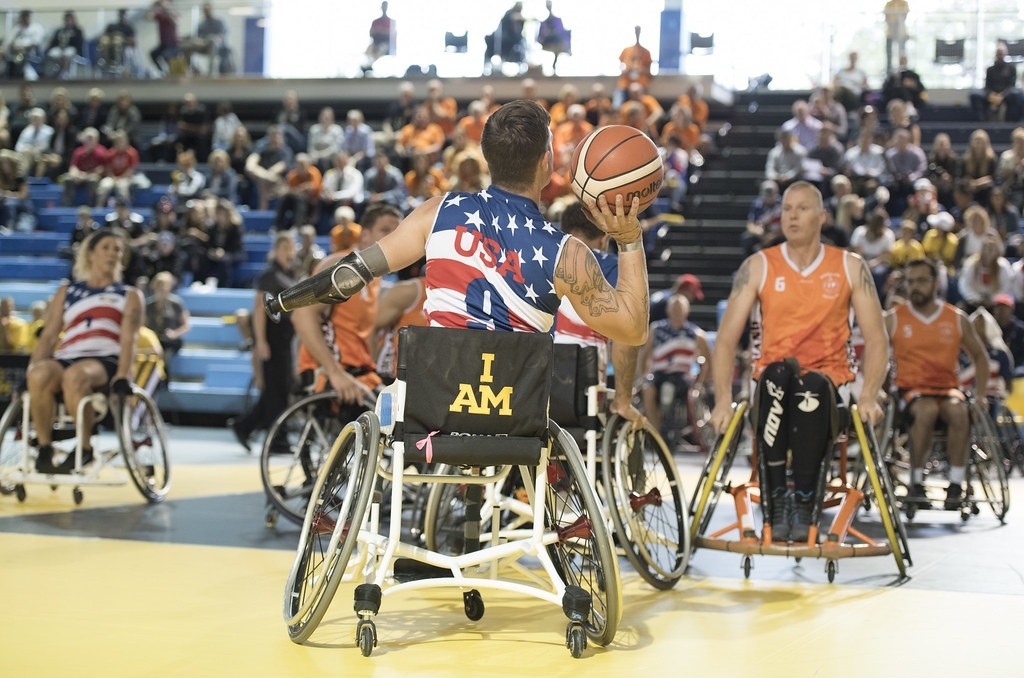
[569, 124, 665, 215]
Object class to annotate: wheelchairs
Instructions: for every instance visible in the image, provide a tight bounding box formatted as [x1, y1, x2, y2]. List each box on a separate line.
[252, 350, 1024, 531]
[412, 342, 692, 590]
[0, 325, 172, 504]
[688, 398, 915, 583]
[286, 325, 624, 658]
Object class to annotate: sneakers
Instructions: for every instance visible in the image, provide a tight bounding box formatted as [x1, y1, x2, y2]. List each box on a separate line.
[381, 478, 469, 525]
[943, 483, 964, 510]
[770, 486, 791, 541]
[790, 489, 814, 541]
[909, 482, 932, 508]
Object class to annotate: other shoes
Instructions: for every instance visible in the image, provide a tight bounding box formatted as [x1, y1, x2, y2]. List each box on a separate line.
[228, 417, 253, 452]
[267, 444, 294, 457]
[58, 446, 93, 469]
[35, 455, 64, 474]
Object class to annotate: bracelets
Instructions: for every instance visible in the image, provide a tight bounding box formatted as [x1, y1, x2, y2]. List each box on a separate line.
[617, 240, 643, 252]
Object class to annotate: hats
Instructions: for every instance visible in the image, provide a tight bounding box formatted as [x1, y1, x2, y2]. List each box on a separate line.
[678, 272, 704, 301]
[992, 294, 1015, 311]
[926, 212, 954, 231]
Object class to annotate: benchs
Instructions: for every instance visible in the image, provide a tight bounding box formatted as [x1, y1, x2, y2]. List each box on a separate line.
[2, 104, 1023, 429]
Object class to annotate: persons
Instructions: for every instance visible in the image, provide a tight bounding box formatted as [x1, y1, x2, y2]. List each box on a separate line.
[483, 2, 526, 76]
[14, 107, 55, 179]
[0, 0, 710, 453]
[884, 259, 990, 509]
[969, 43, 1017, 121]
[26, 228, 146, 474]
[261, 100, 664, 583]
[361, 0, 397, 71]
[882, 0, 910, 76]
[649, 275, 703, 324]
[746, 41, 1024, 398]
[710, 181, 889, 542]
[612, 26, 651, 109]
[536, 0, 573, 74]
